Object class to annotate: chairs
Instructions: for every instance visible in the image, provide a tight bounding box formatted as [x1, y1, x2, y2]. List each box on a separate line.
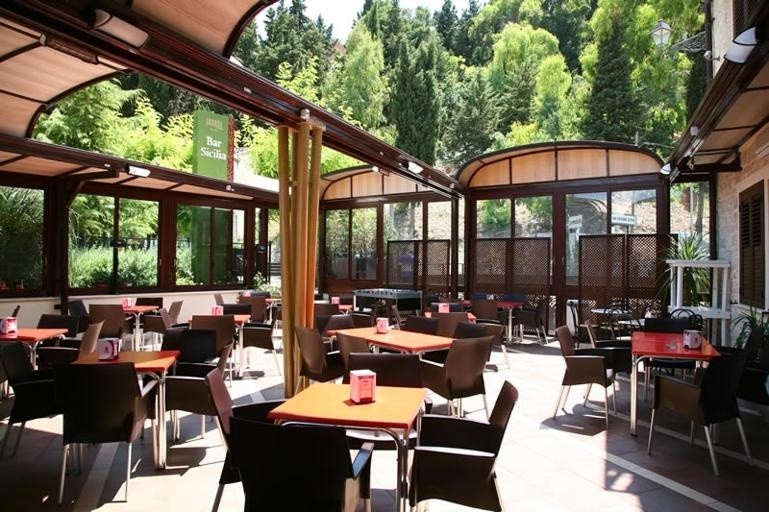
[1, 341, 64, 457]
[205, 368, 287, 512]
[420, 335, 495, 419]
[1, 289, 769, 388]
[409, 380, 518, 509]
[228, 416, 376, 512]
[59, 360, 159, 505]
[646, 350, 752, 478]
[142, 337, 236, 469]
[716, 368, 769, 445]
[556, 326, 617, 429]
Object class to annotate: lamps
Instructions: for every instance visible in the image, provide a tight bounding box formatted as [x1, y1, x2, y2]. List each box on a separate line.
[126, 163, 152, 178]
[79, 6, 152, 50]
[659, 162, 674, 176]
[648, 17, 706, 54]
[396, 153, 425, 176]
[722, 25, 759, 66]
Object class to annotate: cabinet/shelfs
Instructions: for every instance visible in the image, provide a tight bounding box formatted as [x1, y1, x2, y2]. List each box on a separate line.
[663, 257, 733, 348]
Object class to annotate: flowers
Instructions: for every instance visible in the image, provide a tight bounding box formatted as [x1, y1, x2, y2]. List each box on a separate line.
[252, 269, 282, 297]
